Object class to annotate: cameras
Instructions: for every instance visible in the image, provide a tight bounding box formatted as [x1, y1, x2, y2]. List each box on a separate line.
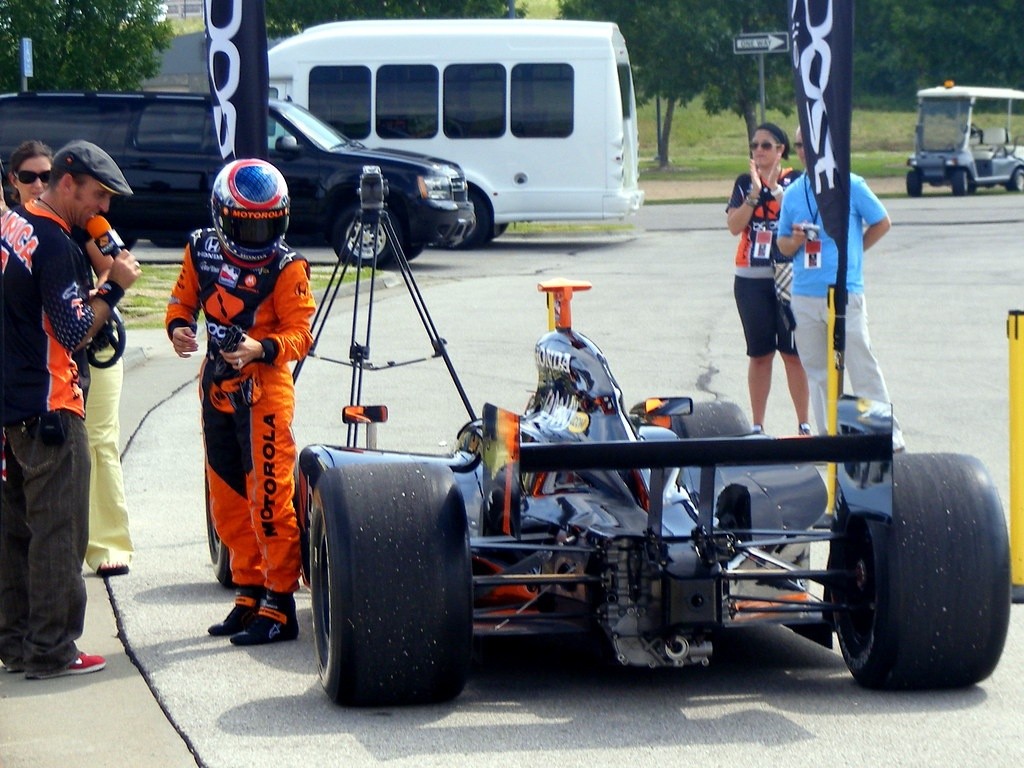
[357, 165, 389, 209]
[801, 223, 820, 242]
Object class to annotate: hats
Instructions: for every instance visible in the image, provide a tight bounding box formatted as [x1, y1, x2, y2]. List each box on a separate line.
[756, 122, 791, 158]
[51, 140, 135, 197]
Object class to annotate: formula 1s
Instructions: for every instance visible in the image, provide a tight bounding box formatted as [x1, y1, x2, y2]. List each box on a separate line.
[206, 274, 1024, 708]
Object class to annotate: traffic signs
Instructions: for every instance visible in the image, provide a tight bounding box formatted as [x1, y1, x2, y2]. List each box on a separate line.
[733, 32, 789, 53]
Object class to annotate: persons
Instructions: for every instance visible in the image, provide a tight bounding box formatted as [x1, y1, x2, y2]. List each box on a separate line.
[725, 122, 814, 436]
[165, 158, 316, 648]
[776, 123, 908, 454]
[0, 139, 145, 680]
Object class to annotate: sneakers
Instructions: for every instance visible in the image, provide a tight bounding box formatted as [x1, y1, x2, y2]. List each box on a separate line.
[7, 649, 108, 680]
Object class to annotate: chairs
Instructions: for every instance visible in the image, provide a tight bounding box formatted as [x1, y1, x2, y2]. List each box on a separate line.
[140, 108, 175, 142]
[970, 127, 1012, 159]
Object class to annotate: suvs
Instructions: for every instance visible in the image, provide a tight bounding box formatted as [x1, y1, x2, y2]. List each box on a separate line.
[0, 87, 474, 268]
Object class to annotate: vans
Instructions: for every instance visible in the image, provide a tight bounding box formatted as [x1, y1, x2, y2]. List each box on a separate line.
[268, 18, 645, 250]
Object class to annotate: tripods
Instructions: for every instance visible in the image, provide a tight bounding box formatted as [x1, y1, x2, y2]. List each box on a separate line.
[292, 209, 479, 449]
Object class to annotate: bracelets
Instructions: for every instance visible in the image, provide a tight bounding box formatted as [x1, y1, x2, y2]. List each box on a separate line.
[96, 279, 125, 311]
[744, 195, 759, 206]
[771, 184, 784, 197]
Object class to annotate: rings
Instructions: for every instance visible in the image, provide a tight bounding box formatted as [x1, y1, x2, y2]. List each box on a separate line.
[237, 358, 244, 367]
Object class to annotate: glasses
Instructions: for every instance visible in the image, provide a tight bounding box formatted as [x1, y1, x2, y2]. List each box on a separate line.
[13, 168, 54, 186]
[747, 140, 780, 150]
[793, 142, 803, 149]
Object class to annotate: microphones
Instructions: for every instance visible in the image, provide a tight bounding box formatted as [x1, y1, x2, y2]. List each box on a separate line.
[88, 216, 124, 259]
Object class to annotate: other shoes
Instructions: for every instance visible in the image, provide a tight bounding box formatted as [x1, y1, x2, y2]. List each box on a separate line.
[208, 584, 299, 644]
[751, 425, 765, 435]
[799, 423, 812, 436]
[96, 562, 130, 578]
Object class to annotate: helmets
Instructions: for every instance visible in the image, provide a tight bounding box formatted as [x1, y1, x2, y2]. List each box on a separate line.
[211, 158, 291, 270]
